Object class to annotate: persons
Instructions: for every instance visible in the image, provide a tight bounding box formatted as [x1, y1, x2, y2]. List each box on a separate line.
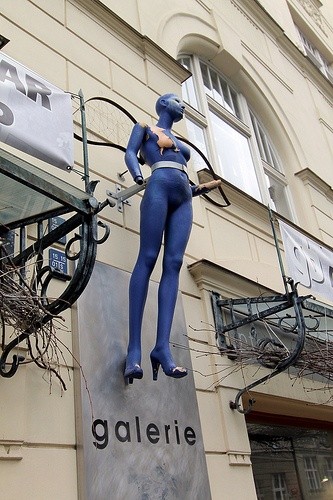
[121, 93, 194, 385]
